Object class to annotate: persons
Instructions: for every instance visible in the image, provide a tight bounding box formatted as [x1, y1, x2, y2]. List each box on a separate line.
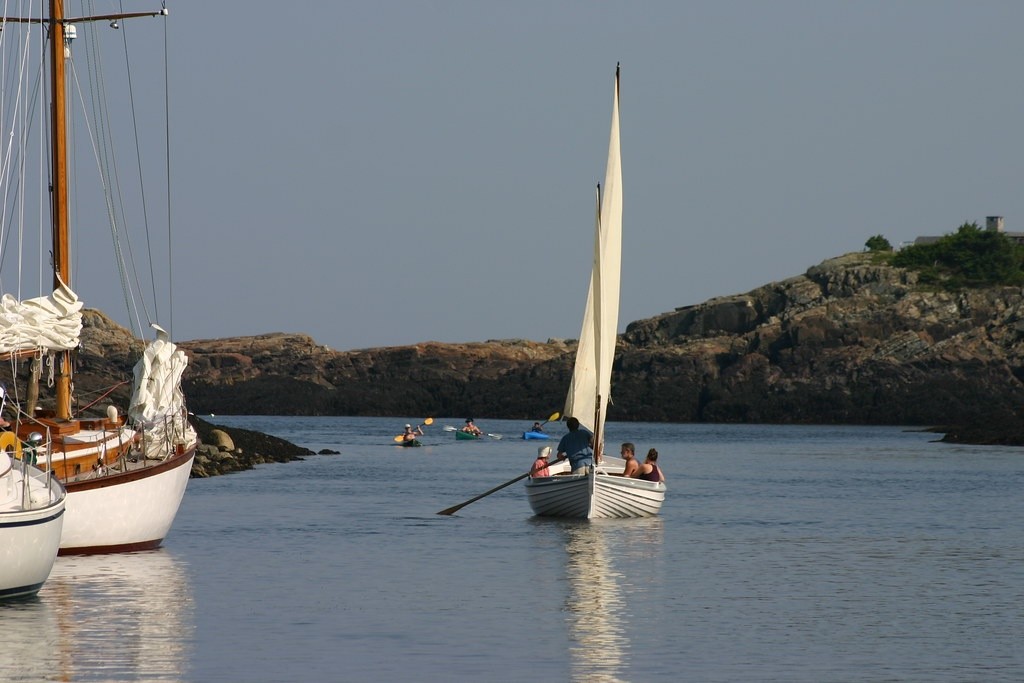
[531, 446, 552, 478]
[557, 417, 594, 476]
[630, 448, 665, 483]
[401, 423, 425, 441]
[620, 443, 642, 477]
[462, 418, 481, 437]
[532, 422, 542, 432]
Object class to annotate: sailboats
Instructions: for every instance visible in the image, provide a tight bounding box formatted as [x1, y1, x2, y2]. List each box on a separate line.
[0, 0, 199, 555]
[524, 58, 668, 520]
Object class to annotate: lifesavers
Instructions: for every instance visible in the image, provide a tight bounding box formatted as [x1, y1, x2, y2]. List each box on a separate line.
[0, 431, 22, 459]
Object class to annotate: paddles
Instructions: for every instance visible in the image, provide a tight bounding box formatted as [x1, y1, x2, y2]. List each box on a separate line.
[540, 412, 560, 426]
[394, 418, 433, 442]
[443, 424, 503, 440]
[437, 455, 568, 515]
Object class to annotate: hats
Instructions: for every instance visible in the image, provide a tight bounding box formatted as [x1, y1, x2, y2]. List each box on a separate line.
[405, 424, 411, 427]
[465, 417, 473, 423]
[538, 446, 552, 457]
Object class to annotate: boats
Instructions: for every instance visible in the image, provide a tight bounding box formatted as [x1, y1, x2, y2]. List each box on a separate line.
[523, 431, 549, 440]
[402, 439, 423, 446]
[0, 395, 68, 604]
[455, 429, 479, 440]
